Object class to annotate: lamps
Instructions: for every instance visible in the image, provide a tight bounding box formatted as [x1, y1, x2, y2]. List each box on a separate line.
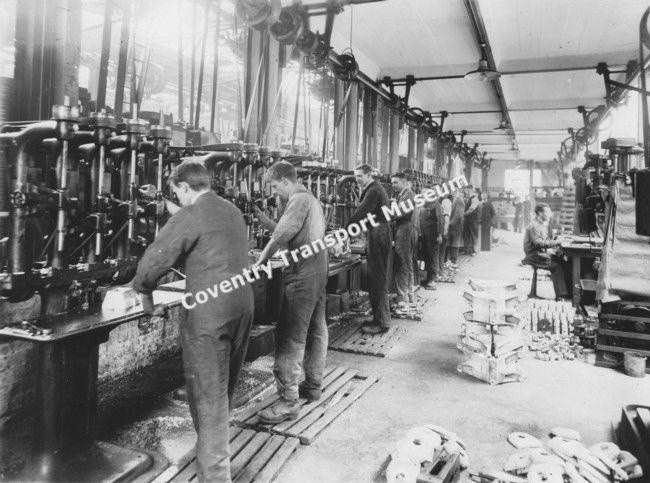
[464, 43, 502, 83]
[493, 119, 512, 133]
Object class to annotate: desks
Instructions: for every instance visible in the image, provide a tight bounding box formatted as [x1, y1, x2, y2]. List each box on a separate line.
[1, 234, 367, 483]
[561, 241, 602, 307]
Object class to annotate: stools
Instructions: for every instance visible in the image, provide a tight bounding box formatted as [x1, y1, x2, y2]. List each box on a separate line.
[522, 259, 548, 298]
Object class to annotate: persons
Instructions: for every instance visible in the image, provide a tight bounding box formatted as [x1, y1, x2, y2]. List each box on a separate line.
[249, 160, 331, 426]
[128, 161, 257, 483]
[521, 200, 572, 302]
[333, 163, 533, 336]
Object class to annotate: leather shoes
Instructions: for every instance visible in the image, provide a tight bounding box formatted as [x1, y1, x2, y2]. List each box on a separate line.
[256, 397, 299, 424]
[299, 381, 322, 400]
[361, 324, 388, 334]
